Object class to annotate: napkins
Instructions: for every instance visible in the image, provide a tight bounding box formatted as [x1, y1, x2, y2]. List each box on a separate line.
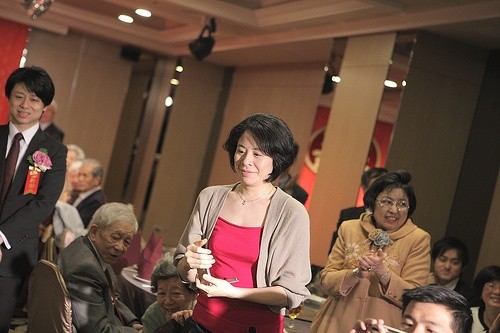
[138, 230, 165, 278]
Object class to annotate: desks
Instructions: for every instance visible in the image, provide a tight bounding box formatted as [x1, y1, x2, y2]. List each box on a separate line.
[121, 265, 319, 333]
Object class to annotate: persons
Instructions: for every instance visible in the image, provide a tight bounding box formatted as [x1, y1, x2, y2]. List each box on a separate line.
[472, 265, 500, 333]
[328, 166, 390, 259]
[173, 113, 312, 333]
[309, 171, 432, 333]
[428, 236, 471, 308]
[141, 248, 198, 333]
[349, 285, 475, 333]
[271, 164, 308, 206]
[40, 142, 106, 257]
[0, 66, 68, 333]
[55, 202, 143, 333]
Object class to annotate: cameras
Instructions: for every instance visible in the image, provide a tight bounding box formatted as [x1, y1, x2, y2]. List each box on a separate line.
[369, 324, 405, 333]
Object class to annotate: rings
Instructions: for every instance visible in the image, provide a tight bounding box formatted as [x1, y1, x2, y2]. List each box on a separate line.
[368, 266, 373, 271]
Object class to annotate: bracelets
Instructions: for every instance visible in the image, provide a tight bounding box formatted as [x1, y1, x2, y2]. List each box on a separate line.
[377, 268, 390, 278]
[353, 268, 361, 280]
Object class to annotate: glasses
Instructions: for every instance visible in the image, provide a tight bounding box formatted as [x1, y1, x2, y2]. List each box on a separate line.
[376, 197, 410, 211]
[485, 281, 500, 291]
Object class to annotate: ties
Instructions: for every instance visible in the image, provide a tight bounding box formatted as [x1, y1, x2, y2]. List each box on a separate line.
[0, 133, 24, 212]
[105, 268, 124, 326]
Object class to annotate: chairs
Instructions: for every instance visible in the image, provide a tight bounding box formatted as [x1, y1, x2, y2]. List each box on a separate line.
[29, 232, 75, 333]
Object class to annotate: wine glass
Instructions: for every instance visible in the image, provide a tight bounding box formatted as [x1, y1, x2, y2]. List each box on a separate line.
[286, 304, 303, 329]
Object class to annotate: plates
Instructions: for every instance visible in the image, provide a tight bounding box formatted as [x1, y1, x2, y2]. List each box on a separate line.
[133, 274, 151, 284]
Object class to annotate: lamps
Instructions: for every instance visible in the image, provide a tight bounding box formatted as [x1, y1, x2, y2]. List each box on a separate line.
[22, 0, 54, 21]
[188, 17, 216, 61]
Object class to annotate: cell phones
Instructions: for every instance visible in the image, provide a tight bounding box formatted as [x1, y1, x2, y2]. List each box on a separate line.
[197, 268, 211, 284]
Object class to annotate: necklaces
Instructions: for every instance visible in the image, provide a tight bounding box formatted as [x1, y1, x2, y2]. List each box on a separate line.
[238, 188, 273, 205]
